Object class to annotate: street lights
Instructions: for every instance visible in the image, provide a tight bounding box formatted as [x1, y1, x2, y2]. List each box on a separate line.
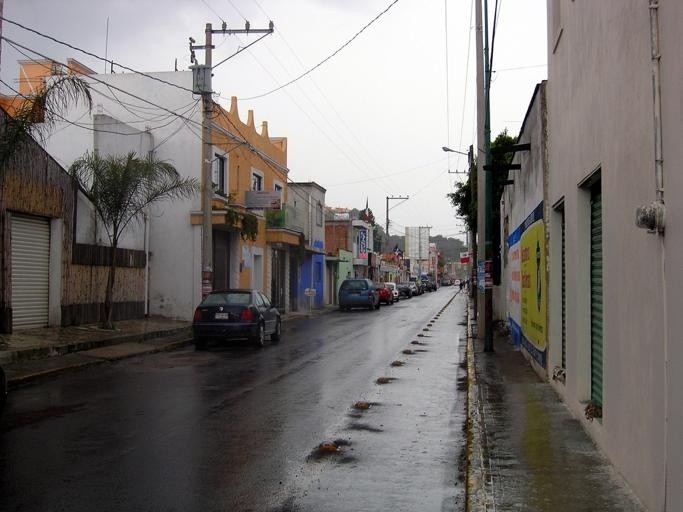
[435, 140, 476, 276]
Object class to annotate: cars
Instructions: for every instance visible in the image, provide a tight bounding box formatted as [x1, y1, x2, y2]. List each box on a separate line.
[189, 287, 282, 351]
[336, 274, 465, 312]
